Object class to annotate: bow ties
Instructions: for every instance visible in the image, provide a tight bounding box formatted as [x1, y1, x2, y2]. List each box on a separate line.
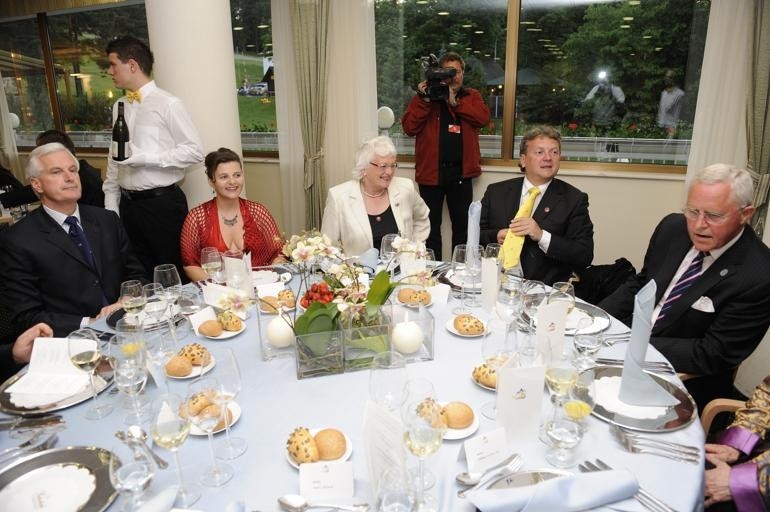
[125, 90, 140, 104]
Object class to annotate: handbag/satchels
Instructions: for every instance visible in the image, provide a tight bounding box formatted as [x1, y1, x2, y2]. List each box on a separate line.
[568, 257, 636, 305]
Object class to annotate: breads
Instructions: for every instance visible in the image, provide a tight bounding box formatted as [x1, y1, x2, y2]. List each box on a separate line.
[278, 289, 296, 308]
[165, 356, 193, 376]
[197, 319, 221, 336]
[419, 399, 446, 429]
[217, 311, 242, 331]
[472, 364, 497, 389]
[441, 401, 473, 429]
[454, 314, 483, 335]
[288, 428, 318, 464]
[411, 290, 431, 306]
[198, 403, 232, 432]
[314, 429, 346, 461]
[398, 288, 417, 301]
[176, 389, 215, 416]
[177, 342, 210, 366]
[259, 296, 280, 311]
[485, 354, 509, 367]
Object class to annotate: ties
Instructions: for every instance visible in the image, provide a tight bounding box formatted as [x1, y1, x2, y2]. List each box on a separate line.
[64, 217, 109, 307]
[651, 251, 711, 336]
[497, 187, 541, 269]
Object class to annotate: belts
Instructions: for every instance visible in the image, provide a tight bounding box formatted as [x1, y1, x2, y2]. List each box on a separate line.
[120, 185, 177, 201]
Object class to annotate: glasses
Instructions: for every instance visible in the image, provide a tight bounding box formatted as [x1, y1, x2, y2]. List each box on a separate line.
[682, 207, 744, 225]
[370, 162, 397, 170]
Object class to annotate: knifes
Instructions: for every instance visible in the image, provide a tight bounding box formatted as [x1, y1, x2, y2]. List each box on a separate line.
[578, 457, 680, 511]
[1, 419, 62, 431]
[593, 357, 675, 376]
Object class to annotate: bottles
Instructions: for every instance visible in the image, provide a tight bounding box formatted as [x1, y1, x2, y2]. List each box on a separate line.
[112, 102, 129, 160]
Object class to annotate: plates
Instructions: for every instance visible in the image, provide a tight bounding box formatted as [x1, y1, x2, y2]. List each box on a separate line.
[341, 255, 400, 277]
[107, 298, 193, 333]
[396, 287, 433, 308]
[0, 345, 119, 417]
[185, 306, 248, 340]
[476, 472, 572, 512]
[371, 250, 400, 277]
[446, 311, 492, 338]
[178, 388, 241, 437]
[164, 340, 216, 379]
[522, 290, 611, 337]
[285, 426, 355, 471]
[260, 287, 299, 314]
[470, 351, 509, 392]
[0, 443, 124, 511]
[574, 366, 696, 434]
[426, 397, 479, 442]
[252, 264, 294, 289]
[439, 265, 489, 293]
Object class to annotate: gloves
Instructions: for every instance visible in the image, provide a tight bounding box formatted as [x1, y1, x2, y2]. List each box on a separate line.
[105, 206, 120, 218]
[112, 141, 145, 168]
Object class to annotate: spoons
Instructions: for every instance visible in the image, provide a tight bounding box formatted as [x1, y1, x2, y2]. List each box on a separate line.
[278, 492, 373, 511]
[428, 258, 455, 277]
[124, 424, 170, 472]
[457, 455, 518, 486]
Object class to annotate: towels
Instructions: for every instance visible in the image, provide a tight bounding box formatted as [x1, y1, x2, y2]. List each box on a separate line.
[467, 470, 640, 512]
[7, 336, 95, 408]
[465, 200, 484, 280]
[199, 280, 254, 321]
[619, 278, 681, 408]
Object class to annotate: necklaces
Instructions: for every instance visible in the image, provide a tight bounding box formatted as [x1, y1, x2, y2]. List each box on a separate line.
[360, 180, 386, 198]
[216, 197, 240, 226]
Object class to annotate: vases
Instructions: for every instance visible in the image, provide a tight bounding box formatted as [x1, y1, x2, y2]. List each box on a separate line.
[337, 307, 391, 372]
[291, 326, 346, 380]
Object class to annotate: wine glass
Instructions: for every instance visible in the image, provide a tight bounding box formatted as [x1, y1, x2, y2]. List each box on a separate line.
[199, 248, 222, 285]
[67, 329, 113, 421]
[114, 360, 149, 425]
[380, 232, 403, 283]
[378, 469, 415, 512]
[115, 314, 145, 349]
[186, 376, 233, 487]
[538, 358, 577, 447]
[399, 376, 441, 488]
[549, 403, 584, 471]
[571, 381, 597, 435]
[150, 391, 201, 509]
[466, 244, 483, 307]
[109, 436, 157, 512]
[485, 242, 505, 303]
[118, 278, 147, 332]
[223, 249, 244, 257]
[154, 263, 184, 349]
[141, 284, 169, 356]
[480, 317, 519, 424]
[370, 351, 411, 415]
[573, 315, 603, 371]
[550, 281, 575, 341]
[451, 243, 472, 316]
[416, 248, 436, 286]
[400, 406, 444, 512]
[520, 279, 548, 356]
[502, 267, 524, 309]
[108, 332, 151, 411]
[202, 346, 248, 462]
[207, 251, 223, 289]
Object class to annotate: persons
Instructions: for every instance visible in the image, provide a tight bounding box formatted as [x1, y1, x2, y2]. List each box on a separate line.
[479, 127, 594, 288]
[179, 146, 288, 282]
[315, 133, 432, 260]
[98, 33, 205, 282]
[655, 69, 686, 130]
[700, 372, 770, 511]
[401, 49, 492, 261]
[582, 65, 627, 129]
[594, 160, 769, 410]
[0, 128, 151, 381]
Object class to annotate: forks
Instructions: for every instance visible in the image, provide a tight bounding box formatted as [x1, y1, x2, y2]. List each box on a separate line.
[608, 424, 703, 466]
[116, 430, 154, 479]
[73, 326, 117, 338]
[0, 426, 60, 468]
[457, 457, 524, 498]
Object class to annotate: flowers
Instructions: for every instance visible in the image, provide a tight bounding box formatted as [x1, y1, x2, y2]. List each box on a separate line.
[274, 226, 429, 366]
